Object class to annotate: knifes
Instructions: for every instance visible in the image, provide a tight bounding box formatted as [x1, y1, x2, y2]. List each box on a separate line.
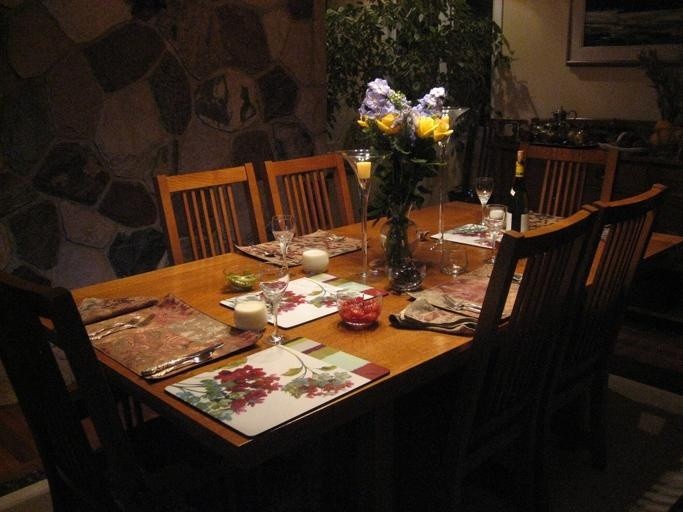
[139, 344, 225, 377]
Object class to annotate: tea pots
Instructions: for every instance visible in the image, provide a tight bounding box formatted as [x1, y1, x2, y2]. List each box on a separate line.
[545, 106, 579, 141]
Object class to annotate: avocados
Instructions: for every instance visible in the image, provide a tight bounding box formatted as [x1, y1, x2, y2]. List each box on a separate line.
[617, 132, 646, 148]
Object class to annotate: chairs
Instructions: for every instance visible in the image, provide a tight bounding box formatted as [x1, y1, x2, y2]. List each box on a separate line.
[444, 118, 520, 205]
[506, 179, 667, 469]
[261, 152, 355, 240]
[154, 163, 269, 266]
[399, 205, 599, 507]
[0, 270, 215, 510]
[512, 141, 619, 222]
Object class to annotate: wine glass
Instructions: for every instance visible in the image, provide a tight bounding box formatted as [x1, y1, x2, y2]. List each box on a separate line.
[337, 148, 393, 285]
[271, 214, 298, 273]
[425, 104, 467, 262]
[482, 203, 506, 265]
[474, 175, 495, 226]
[260, 260, 292, 346]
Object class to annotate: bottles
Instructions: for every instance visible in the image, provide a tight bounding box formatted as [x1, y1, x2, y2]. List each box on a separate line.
[507, 148, 531, 234]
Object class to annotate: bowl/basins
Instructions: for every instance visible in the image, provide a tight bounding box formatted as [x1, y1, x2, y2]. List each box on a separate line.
[335, 287, 384, 330]
[222, 261, 258, 292]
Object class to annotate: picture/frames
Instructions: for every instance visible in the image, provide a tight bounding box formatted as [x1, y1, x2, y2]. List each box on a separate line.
[565, 0, 683, 68]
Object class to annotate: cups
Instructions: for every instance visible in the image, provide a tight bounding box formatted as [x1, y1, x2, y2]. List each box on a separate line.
[440, 243, 468, 276]
[301, 237, 330, 275]
[657, 127, 683, 161]
[232, 294, 267, 336]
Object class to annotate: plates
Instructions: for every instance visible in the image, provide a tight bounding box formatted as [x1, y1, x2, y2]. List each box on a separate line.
[599, 141, 648, 156]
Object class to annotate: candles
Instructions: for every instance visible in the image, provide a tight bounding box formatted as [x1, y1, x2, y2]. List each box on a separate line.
[356, 161, 371, 179]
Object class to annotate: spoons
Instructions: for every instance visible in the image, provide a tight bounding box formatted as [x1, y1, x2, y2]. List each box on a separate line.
[147, 348, 217, 380]
[84, 313, 155, 342]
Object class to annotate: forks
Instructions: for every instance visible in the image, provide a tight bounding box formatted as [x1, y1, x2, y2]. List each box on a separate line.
[441, 294, 510, 320]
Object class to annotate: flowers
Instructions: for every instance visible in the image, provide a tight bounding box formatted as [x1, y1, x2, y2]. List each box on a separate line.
[356, 76, 455, 265]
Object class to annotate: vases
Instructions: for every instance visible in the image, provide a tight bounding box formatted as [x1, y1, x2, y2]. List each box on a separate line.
[379, 201, 418, 274]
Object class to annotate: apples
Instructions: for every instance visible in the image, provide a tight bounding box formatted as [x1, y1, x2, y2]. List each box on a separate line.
[649, 120, 683, 146]
[567, 130, 602, 144]
